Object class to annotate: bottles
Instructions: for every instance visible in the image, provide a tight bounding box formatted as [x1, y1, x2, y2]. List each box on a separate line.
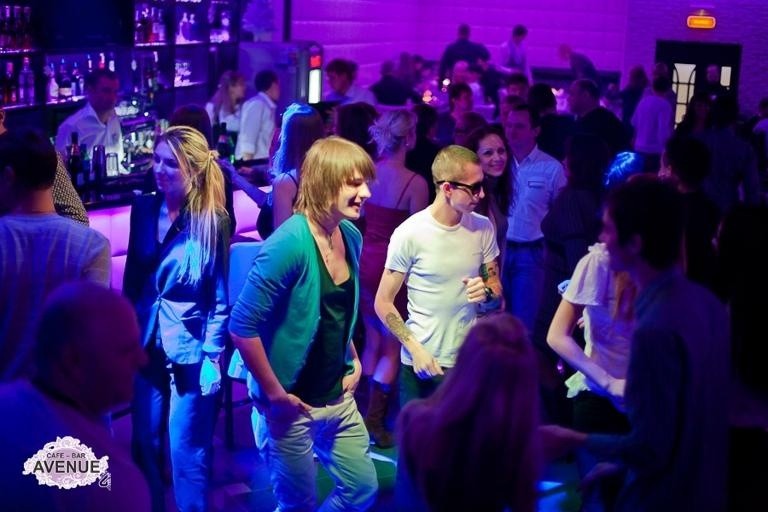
[2, 62, 17, 103]
[47, 53, 106, 100]
[216, 121, 234, 166]
[134, 6, 167, 42]
[18, 57, 36, 103]
[210, 11, 229, 40]
[124, 129, 154, 170]
[129, 93, 157, 118]
[180, 11, 196, 41]
[1, 5, 33, 53]
[145, 51, 161, 87]
[64, 132, 119, 206]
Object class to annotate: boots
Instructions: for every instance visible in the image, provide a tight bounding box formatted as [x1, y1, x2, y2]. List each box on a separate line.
[365, 378, 393, 450]
[354, 373, 372, 419]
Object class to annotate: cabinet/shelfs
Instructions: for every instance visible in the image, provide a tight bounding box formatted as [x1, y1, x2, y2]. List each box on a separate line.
[2, 0, 242, 170]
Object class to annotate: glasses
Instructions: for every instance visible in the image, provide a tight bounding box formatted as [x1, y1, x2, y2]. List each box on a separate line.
[437, 174, 488, 195]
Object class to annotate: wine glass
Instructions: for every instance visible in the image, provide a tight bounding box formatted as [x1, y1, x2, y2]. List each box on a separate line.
[175, 60, 191, 85]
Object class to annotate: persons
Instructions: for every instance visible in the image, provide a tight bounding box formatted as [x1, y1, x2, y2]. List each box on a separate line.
[0, 20, 768, 511]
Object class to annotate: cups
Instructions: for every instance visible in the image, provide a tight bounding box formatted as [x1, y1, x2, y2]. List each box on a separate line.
[422, 81, 449, 105]
